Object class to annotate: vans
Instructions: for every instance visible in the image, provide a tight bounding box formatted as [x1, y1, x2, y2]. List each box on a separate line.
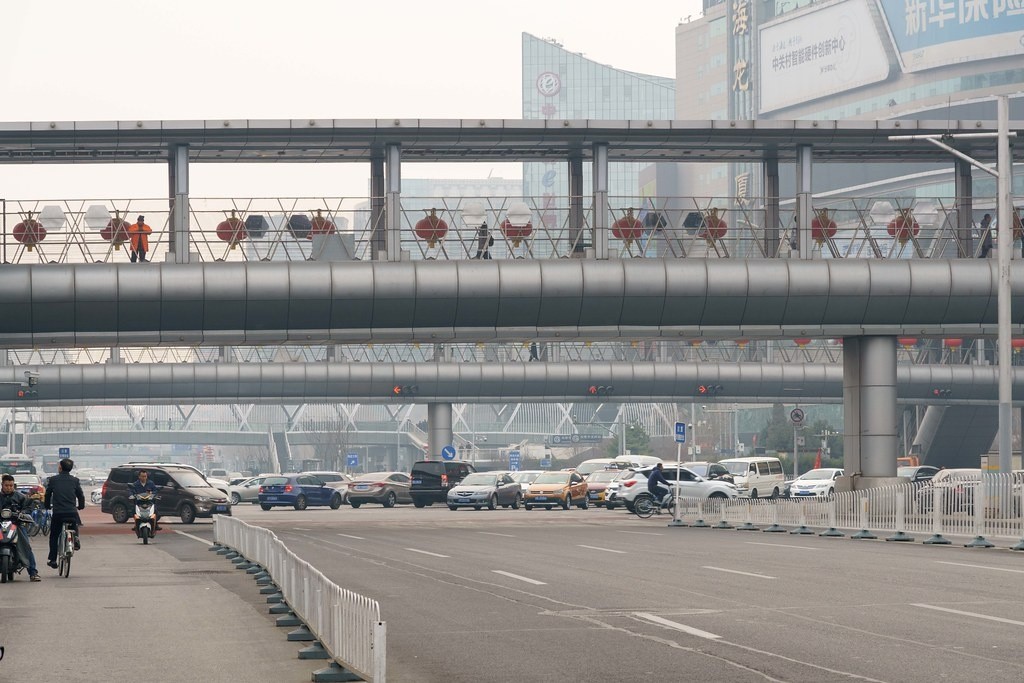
[204, 469, 231, 482]
[718, 457, 786, 499]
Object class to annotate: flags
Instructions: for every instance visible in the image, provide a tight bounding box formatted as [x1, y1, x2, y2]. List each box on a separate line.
[814, 451, 820, 468]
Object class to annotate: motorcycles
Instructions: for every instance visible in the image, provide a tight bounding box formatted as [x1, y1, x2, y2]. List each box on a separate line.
[0, 505, 35, 584]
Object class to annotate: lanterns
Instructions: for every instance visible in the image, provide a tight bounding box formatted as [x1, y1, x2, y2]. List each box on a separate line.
[1012, 339, 1024, 352]
[898, 338, 917, 350]
[945, 339, 962, 352]
[611, 209, 836, 247]
[13, 210, 47, 251]
[501, 219, 532, 247]
[216, 209, 335, 249]
[888, 210, 919, 247]
[735, 340, 749, 349]
[100, 211, 131, 250]
[688, 340, 704, 347]
[794, 339, 811, 348]
[415, 209, 448, 247]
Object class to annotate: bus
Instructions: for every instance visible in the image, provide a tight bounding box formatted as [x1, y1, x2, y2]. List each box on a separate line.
[40, 454, 62, 481]
[0, 454, 37, 478]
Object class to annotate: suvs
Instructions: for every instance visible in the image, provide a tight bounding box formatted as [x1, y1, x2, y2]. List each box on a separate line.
[604, 465, 738, 513]
[409, 460, 477, 508]
[101, 461, 232, 525]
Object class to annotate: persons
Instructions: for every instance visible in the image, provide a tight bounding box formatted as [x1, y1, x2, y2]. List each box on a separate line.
[128, 215, 152, 262]
[140, 416, 175, 430]
[980, 214, 993, 257]
[648, 463, 674, 514]
[529, 343, 539, 362]
[130, 469, 163, 531]
[457, 440, 473, 460]
[789, 215, 797, 248]
[473, 220, 492, 259]
[0, 458, 85, 581]
[417, 418, 428, 431]
[5, 419, 10, 432]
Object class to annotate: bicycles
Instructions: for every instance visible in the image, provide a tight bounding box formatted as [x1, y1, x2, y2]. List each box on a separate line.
[633, 483, 693, 519]
[21, 489, 52, 536]
[50, 505, 84, 579]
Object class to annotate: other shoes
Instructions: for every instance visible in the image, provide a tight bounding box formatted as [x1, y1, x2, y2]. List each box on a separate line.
[155, 524, 162, 531]
[73, 541, 81, 550]
[47, 561, 58, 569]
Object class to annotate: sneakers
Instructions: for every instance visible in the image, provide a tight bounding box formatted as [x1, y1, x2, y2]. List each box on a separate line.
[30, 574, 41, 581]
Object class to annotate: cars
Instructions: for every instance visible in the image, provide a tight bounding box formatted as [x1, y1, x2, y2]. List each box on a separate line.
[12, 475, 45, 495]
[347, 471, 414, 509]
[228, 477, 266, 505]
[521, 471, 591, 511]
[790, 467, 845, 501]
[74, 469, 108, 486]
[577, 455, 683, 509]
[897, 465, 943, 484]
[682, 463, 734, 484]
[916, 468, 986, 515]
[490, 468, 575, 504]
[258, 473, 343, 511]
[300, 471, 355, 506]
[445, 473, 523, 512]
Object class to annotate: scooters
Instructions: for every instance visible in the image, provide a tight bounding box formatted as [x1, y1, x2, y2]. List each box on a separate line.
[127, 481, 162, 545]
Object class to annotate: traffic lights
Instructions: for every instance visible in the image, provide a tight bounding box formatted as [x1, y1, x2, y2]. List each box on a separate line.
[932, 387, 953, 398]
[589, 384, 615, 396]
[393, 386, 417, 398]
[699, 383, 724, 395]
[18, 388, 38, 398]
